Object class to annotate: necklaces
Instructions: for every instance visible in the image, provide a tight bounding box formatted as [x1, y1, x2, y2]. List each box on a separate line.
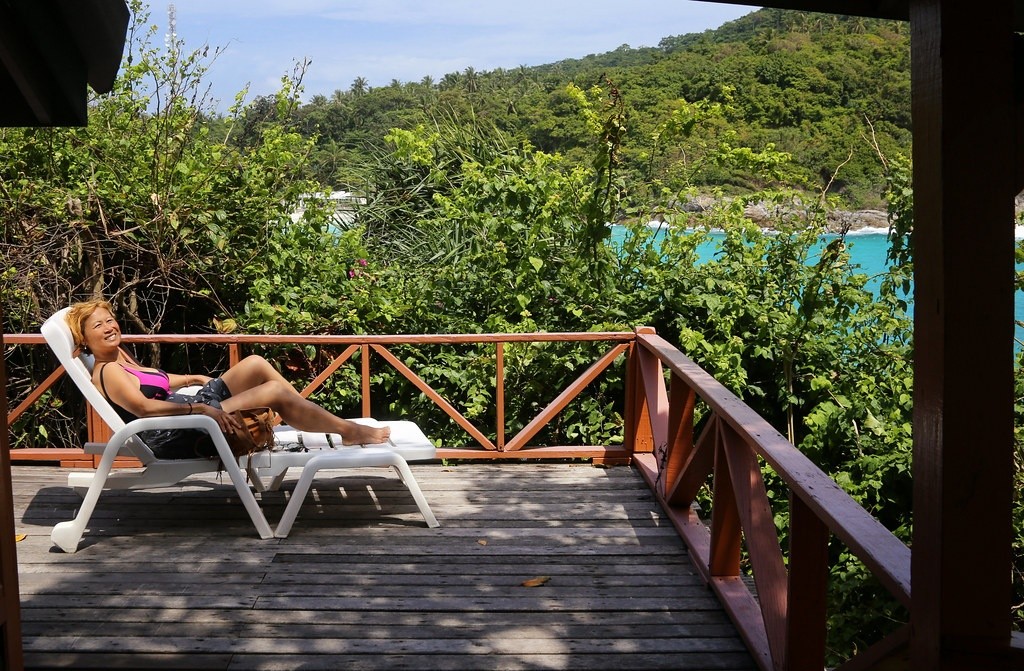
[95, 360, 132, 367]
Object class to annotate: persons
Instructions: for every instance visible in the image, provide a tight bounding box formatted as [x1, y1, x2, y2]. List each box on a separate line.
[69, 300, 389, 459]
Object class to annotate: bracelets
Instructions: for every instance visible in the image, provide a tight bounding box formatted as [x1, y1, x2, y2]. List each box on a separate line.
[187, 402, 193, 415]
[184, 373, 190, 388]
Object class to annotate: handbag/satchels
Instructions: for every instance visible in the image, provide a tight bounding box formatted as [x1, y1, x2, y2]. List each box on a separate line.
[195, 407, 282, 460]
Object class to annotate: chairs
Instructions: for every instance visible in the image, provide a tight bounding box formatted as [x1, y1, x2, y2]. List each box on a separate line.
[39, 308, 444, 553]
